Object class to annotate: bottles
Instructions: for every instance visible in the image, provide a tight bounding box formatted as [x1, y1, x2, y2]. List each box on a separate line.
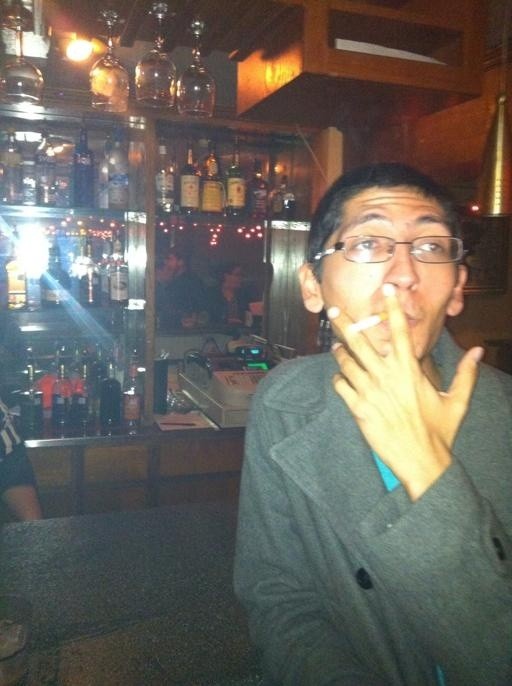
[316, 319, 333, 347]
[6, 339, 143, 431]
[154, 356, 168, 414]
[4, 220, 132, 310]
[157, 132, 301, 218]
[4, 129, 128, 216]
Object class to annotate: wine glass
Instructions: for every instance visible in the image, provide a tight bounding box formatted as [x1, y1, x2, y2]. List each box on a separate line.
[175, 6, 217, 114]
[134, 0, 176, 109]
[89, 4, 129, 112]
[0, 2, 44, 109]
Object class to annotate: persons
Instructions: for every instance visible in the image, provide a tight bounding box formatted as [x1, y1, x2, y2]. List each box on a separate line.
[166, 244, 218, 330]
[0, 303, 45, 530]
[211, 263, 254, 335]
[156, 256, 175, 333]
[227, 156, 512, 684]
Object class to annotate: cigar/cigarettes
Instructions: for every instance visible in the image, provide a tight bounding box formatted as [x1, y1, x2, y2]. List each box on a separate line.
[347, 312, 390, 331]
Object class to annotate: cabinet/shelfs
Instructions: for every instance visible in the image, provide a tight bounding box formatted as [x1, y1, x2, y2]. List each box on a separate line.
[143, 111, 359, 442]
[1, 105, 146, 449]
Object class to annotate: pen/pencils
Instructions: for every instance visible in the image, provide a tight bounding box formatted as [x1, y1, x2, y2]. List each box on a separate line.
[160, 423, 196, 426]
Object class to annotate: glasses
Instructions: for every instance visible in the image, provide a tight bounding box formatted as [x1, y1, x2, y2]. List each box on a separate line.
[310, 234, 470, 266]
